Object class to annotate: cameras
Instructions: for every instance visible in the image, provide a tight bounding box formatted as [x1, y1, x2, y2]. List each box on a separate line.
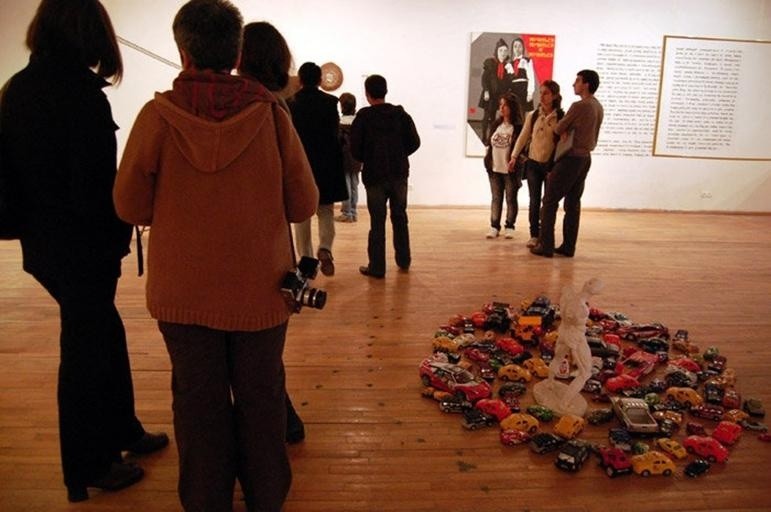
[277, 255, 327, 314]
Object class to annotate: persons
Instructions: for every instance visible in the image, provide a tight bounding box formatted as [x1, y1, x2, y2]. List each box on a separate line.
[507, 80, 565, 249]
[348, 75, 421, 279]
[533, 277, 605, 417]
[478, 39, 514, 147]
[483, 93, 522, 240]
[115, 0, 320, 512]
[334, 92, 360, 223]
[284, 62, 349, 279]
[508, 38, 536, 113]
[530, 70, 604, 257]
[0, 0, 170, 504]
[237, 22, 306, 446]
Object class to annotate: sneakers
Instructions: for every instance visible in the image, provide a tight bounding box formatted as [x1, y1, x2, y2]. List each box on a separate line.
[485, 225, 499, 237]
[553, 244, 565, 256]
[529, 244, 544, 255]
[358, 264, 369, 274]
[317, 246, 335, 278]
[525, 236, 538, 248]
[352, 209, 358, 221]
[503, 225, 513, 239]
[333, 212, 354, 224]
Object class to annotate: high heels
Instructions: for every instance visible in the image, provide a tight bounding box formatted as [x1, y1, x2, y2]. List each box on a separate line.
[66, 465, 144, 504]
[106, 434, 169, 456]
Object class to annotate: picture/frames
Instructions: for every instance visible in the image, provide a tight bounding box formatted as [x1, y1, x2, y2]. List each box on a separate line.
[651, 34, 771, 164]
